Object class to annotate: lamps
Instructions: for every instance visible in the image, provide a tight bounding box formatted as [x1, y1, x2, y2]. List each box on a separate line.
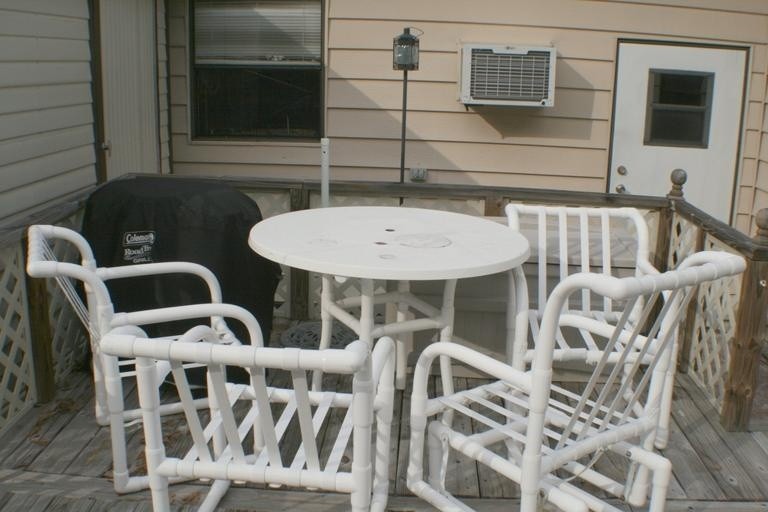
[392, 28, 421, 205]
